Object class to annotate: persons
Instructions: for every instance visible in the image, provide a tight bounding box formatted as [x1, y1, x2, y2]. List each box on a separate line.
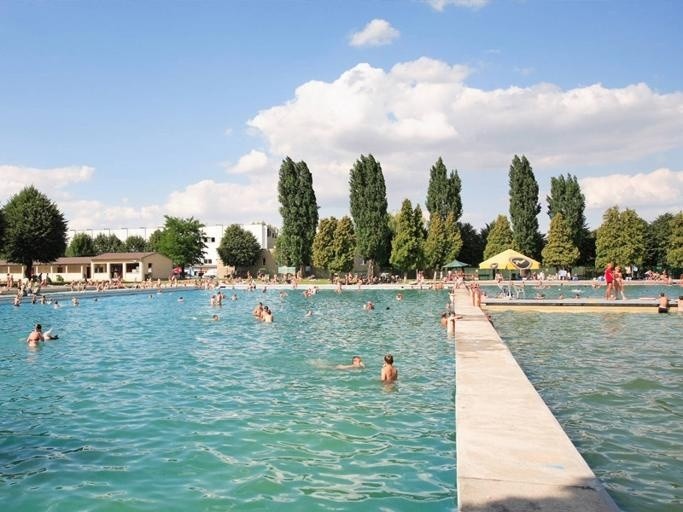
[40, 328, 58, 340]
[379, 353, 397, 383]
[334, 357, 364, 370]
[26, 324, 44, 347]
[1, 263, 683, 328]
[445, 310, 462, 337]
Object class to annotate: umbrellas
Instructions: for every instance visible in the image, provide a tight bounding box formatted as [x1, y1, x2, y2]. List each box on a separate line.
[478, 249, 540, 299]
[441, 259, 468, 274]
